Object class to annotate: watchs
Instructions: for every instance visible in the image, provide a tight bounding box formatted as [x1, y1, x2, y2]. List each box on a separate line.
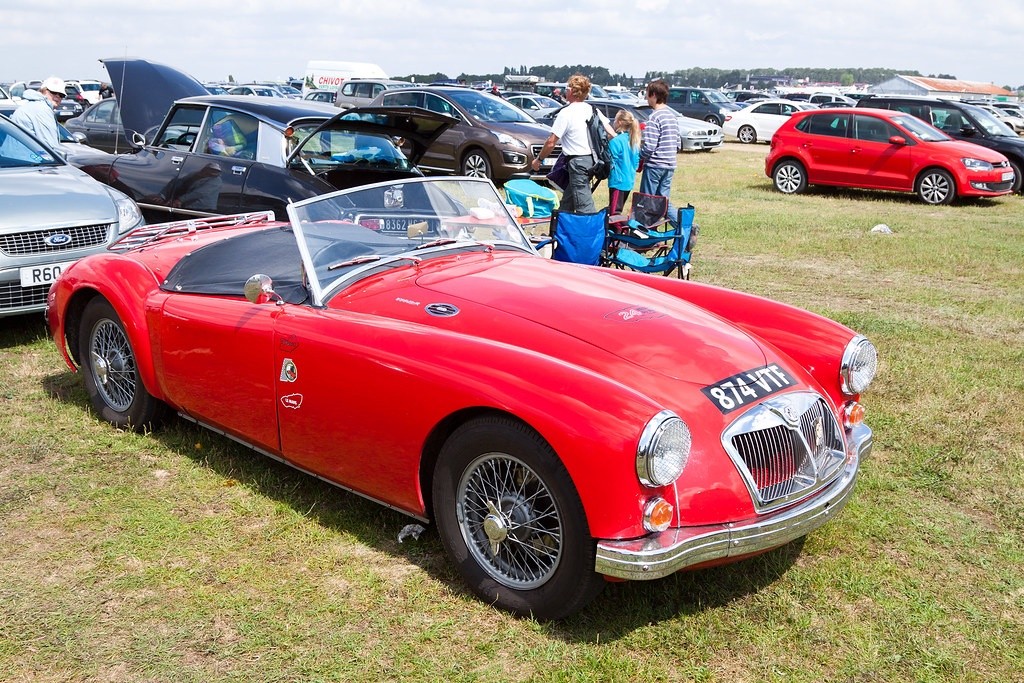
[537, 155, 543, 161]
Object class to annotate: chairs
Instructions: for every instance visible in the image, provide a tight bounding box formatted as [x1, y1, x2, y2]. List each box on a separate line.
[680, 95, 686, 103]
[428, 97, 445, 114]
[944, 112, 964, 131]
[318, 96, 324, 101]
[534, 190, 695, 280]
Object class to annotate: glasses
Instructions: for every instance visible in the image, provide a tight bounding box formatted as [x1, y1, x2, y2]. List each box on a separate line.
[46, 88, 66, 98]
[565, 86, 571, 91]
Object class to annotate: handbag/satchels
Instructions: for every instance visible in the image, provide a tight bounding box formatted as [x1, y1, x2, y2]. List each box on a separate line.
[593, 159, 609, 181]
[586, 105, 611, 163]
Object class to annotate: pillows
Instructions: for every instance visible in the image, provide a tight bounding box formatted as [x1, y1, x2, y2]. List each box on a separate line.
[208, 139, 243, 157]
[212, 119, 247, 150]
[211, 114, 259, 138]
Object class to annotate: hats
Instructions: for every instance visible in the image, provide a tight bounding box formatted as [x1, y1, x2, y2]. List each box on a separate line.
[493, 86, 497, 90]
[43, 76, 67, 97]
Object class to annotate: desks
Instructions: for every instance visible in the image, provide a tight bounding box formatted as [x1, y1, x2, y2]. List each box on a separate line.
[441, 213, 552, 240]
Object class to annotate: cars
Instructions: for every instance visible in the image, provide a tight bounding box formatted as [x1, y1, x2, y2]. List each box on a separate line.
[661, 81, 1023, 145]
[0, 58, 637, 337]
[763, 106, 1016, 208]
[617, 96, 725, 154]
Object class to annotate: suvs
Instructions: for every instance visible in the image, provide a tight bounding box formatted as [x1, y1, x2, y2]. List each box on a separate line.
[856, 93, 1024, 196]
[533, 97, 653, 166]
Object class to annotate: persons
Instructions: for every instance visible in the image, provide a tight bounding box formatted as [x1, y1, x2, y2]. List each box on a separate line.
[98, 82, 114, 100]
[607, 110, 642, 253]
[552, 89, 561, 103]
[490, 86, 501, 97]
[153, 162, 223, 210]
[0, 77, 67, 163]
[638, 88, 646, 99]
[532, 75, 618, 213]
[637, 80, 700, 252]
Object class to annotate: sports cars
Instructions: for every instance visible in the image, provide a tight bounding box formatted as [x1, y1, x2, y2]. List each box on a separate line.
[45, 173, 878, 624]
